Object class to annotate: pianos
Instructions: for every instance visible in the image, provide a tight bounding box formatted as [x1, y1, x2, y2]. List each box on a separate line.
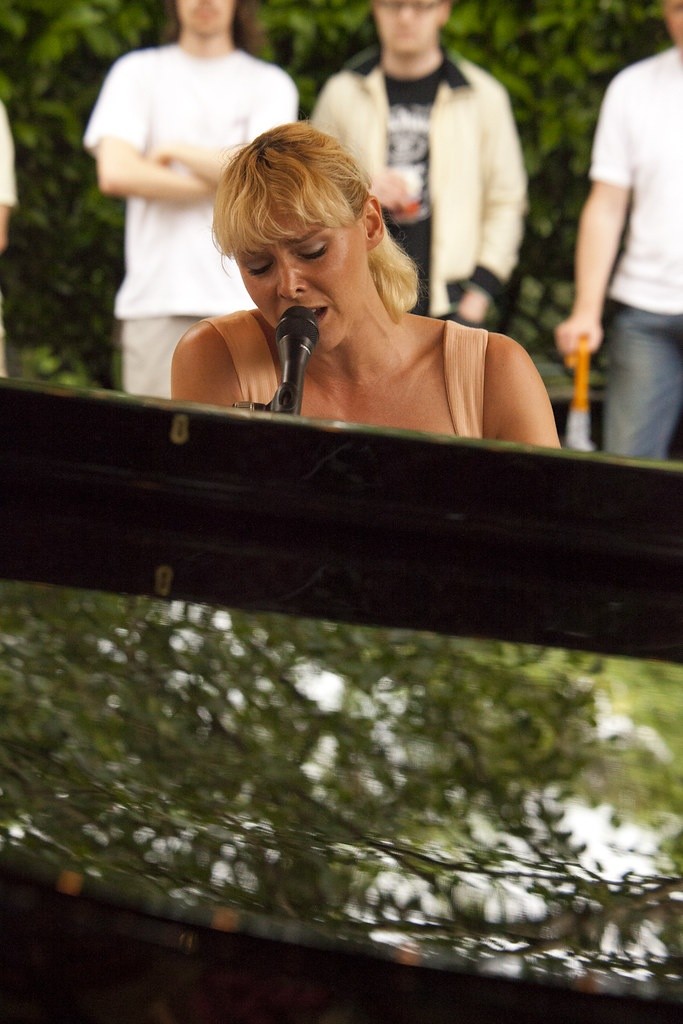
[0, 373, 679, 1023]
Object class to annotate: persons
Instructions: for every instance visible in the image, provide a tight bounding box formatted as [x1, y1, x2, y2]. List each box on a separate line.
[170, 121, 564, 448]
[0, 103, 17, 378]
[555, 0, 683, 463]
[314, 0, 528, 328]
[82, 0, 302, 402]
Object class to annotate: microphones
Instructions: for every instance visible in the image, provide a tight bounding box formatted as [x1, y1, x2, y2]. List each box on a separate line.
[270, 305, 320, 418]
